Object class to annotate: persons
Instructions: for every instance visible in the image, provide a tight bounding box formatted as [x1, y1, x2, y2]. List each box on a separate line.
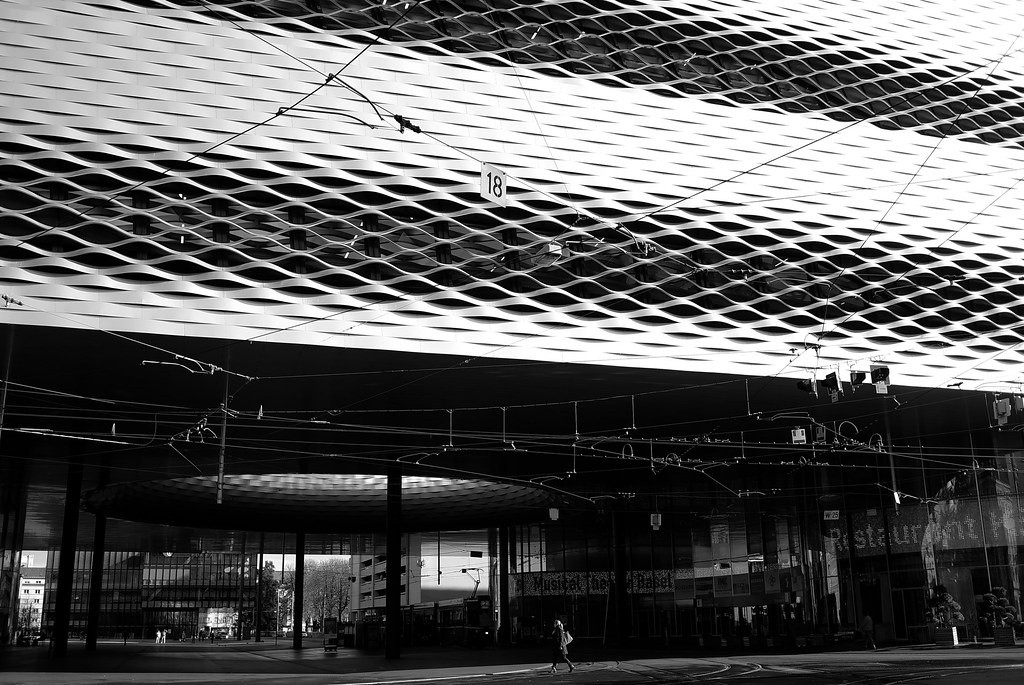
[155, 629, 167, 643]
[857, 611, 877, 651]
[549, 620, 576, 674]
[17, 630, 47, 646]
[121, 629, 130, 646]
[199, 630, 204, 641]
[181, 630, 186, 642]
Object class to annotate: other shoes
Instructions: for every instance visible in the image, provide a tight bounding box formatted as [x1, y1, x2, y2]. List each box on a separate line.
[548, 667, 557, 673]
[569, 664, 575, 672]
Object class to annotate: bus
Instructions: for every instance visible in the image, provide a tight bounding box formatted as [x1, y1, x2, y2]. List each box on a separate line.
[400, 567, 495, 651]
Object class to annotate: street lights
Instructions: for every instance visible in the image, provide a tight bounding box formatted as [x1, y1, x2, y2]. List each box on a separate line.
[276, 589, 281, 645]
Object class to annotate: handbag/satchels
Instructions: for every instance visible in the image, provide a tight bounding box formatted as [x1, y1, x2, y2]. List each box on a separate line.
[563, 631, 573, 645]
